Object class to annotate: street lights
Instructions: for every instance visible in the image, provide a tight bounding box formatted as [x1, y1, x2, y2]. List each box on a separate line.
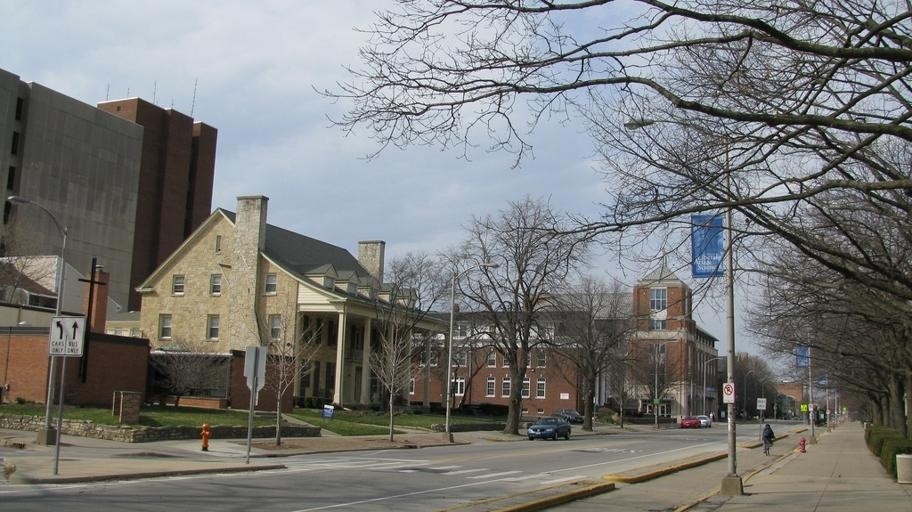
[703, 357, 724, 414]
[653, 340, 681, 425]
[445, 261, 500, 433]
[621, 115, 746, 499]
[7, 195, 71, 449]
[742, 370, 756, 423]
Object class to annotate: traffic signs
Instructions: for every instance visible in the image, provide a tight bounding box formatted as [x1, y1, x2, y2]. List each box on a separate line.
[48, 316, 87, 358]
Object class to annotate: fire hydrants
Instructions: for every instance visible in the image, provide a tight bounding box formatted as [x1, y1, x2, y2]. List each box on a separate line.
[198, 422, 214, 451]
[799, 437, 808, 453]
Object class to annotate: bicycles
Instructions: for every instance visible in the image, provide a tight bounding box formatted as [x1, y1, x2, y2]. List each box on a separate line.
[764, 438, 771, 457]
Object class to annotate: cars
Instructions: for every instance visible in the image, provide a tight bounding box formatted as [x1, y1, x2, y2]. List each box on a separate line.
[557, 408, 585, 424]
[697, 414, 712, 428]
[751, 411, 765, 422]
[680, 415, 702, 429]
[526, 415, 573, 442]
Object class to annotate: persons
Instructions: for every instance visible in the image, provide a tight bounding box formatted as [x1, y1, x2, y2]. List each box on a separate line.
[761, 423, 775, 452]
